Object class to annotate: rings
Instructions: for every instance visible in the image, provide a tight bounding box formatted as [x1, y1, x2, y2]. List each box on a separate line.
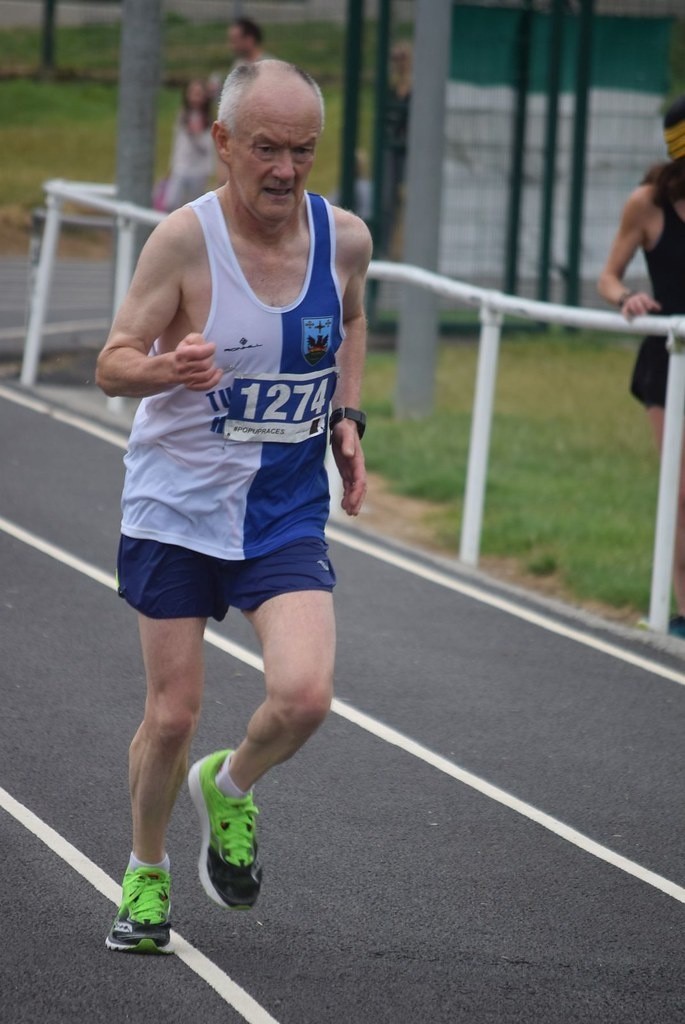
[350, 481, 355, 486]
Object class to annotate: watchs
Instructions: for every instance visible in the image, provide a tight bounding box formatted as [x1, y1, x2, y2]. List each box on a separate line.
[328, 407, 366, 440]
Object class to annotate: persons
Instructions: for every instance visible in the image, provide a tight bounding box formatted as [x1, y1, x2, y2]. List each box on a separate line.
[226, 20, 273, 60]
[155, 76, 216, 216]
[104, 58, 368, 953]
[595, 94, 685, 638]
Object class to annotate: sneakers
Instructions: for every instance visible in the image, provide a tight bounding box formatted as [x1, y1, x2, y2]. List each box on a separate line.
[188, 747, 264, 912]
[105, 865, 174, 954]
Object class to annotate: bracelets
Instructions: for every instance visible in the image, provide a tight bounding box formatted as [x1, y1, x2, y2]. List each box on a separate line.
[618, 291, 637, 305]
